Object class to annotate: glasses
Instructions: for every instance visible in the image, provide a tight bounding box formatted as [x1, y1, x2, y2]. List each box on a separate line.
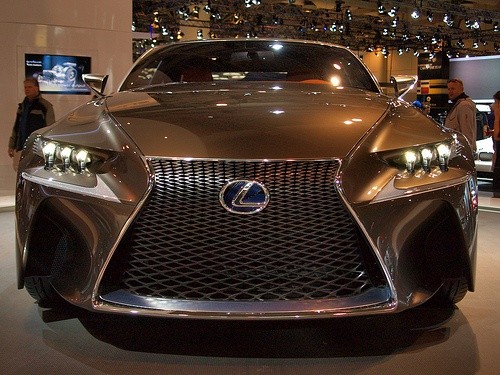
[447, 79, 462, 84]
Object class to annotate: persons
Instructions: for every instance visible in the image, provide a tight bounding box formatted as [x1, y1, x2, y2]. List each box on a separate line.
[421, 102, 440, 121]
[484, 90, 500, 198]
[444, 79, 476, 153]
[8, 77, 55, 174]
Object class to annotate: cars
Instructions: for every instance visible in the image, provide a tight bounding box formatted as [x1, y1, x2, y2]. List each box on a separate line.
[474, 136, 495, 178]
[14, 37, 478, 321]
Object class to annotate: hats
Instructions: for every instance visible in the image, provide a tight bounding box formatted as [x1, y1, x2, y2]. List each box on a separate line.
[413, 101, 421, 107]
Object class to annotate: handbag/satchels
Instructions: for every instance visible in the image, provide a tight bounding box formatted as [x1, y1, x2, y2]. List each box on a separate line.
[477, 112, 488, 139]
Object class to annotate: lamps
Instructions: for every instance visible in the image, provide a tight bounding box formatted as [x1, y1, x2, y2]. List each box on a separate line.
[131, 0, 500, 70]
